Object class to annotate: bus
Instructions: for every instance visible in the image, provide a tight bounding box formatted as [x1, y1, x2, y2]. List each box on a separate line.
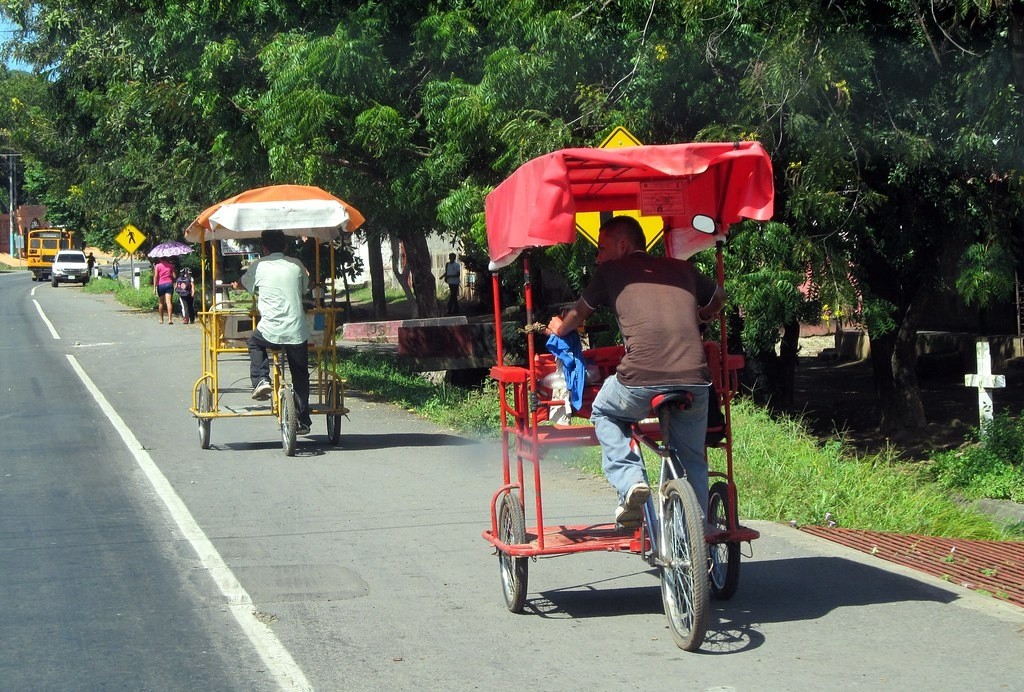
[27, 229, 85, 281]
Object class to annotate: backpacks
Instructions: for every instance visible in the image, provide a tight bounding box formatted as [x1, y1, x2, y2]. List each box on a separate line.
[176, 277, 191, 295]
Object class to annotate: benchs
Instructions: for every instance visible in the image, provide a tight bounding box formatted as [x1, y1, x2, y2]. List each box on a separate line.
[489, 340, 744, 448]
[196, 308, 342, 353]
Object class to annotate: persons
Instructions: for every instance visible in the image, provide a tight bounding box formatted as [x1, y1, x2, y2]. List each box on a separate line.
[112, 258, 119, 278]
[85, 252, 96, 276]
[174, 268, 195, 324]
[548, 215, 722, 596]
[230, 229, 312, 432]
[439, 252, 461, 314]
[154, 257, 177, 324]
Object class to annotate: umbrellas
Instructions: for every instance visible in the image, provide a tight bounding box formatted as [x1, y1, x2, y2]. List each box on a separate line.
[147, 241, 194, 258]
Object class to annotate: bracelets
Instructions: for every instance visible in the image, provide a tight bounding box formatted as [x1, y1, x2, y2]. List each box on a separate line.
[698, 307, 714, 324]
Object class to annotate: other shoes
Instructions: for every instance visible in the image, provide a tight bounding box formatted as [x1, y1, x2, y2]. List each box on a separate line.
[447, 309, 452, 314]
[251, 379, 272, 399]
[183, 317, 188, 324]
[159, 320, 162, 324]
[168, 320, 173, 324]
[298, 417, 312, 431]
[452, 311, 459, 315]
[618, 483, 650, 535]
[190, 317, 194, 323]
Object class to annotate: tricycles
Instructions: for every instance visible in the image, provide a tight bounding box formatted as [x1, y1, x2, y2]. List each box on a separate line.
[482, 123, 775, 652]
[184, 184, 368, 456]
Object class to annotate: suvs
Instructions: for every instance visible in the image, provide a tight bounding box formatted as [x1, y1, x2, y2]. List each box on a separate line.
[51, 251, 90, 288]
